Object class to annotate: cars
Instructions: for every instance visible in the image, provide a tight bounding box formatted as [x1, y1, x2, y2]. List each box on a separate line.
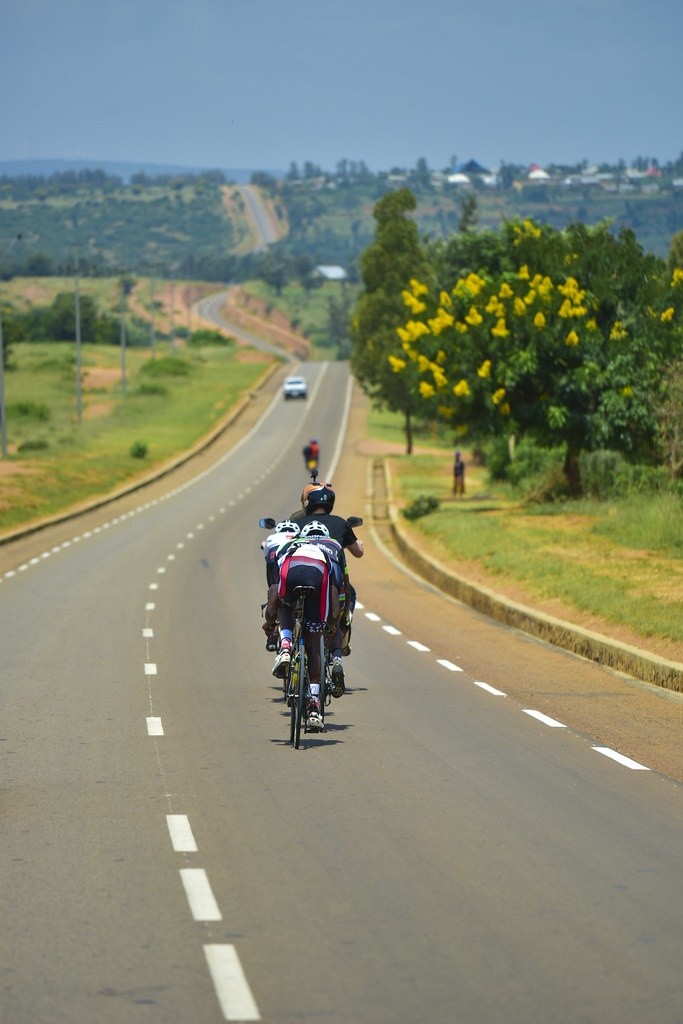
[282, 376, 307, 401]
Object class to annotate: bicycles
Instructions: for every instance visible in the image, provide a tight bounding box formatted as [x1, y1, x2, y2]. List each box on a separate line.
[261, 583, 323, 750]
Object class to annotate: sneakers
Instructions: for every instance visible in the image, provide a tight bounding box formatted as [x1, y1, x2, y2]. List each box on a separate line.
[271, 648, 292, 677]
[306, 704, 324, 728]
[265, 630, 278, 652]
[330, 663, 345, 698]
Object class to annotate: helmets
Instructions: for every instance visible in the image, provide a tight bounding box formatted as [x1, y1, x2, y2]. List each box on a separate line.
[301, 482, 335, 516]
[275, 520, 300, 533]
[311, 439, 318, 444]
[299, 520, 330, 538]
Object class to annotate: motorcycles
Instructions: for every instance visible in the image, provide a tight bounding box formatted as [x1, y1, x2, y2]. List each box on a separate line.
[257, 515, 364, 655]
[307, 460, 316, 472]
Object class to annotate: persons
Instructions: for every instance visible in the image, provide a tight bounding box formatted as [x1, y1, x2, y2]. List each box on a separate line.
[452, 452, 465, 496]
[260, 482, 364, 731]
[310, 439, 319, 461]
